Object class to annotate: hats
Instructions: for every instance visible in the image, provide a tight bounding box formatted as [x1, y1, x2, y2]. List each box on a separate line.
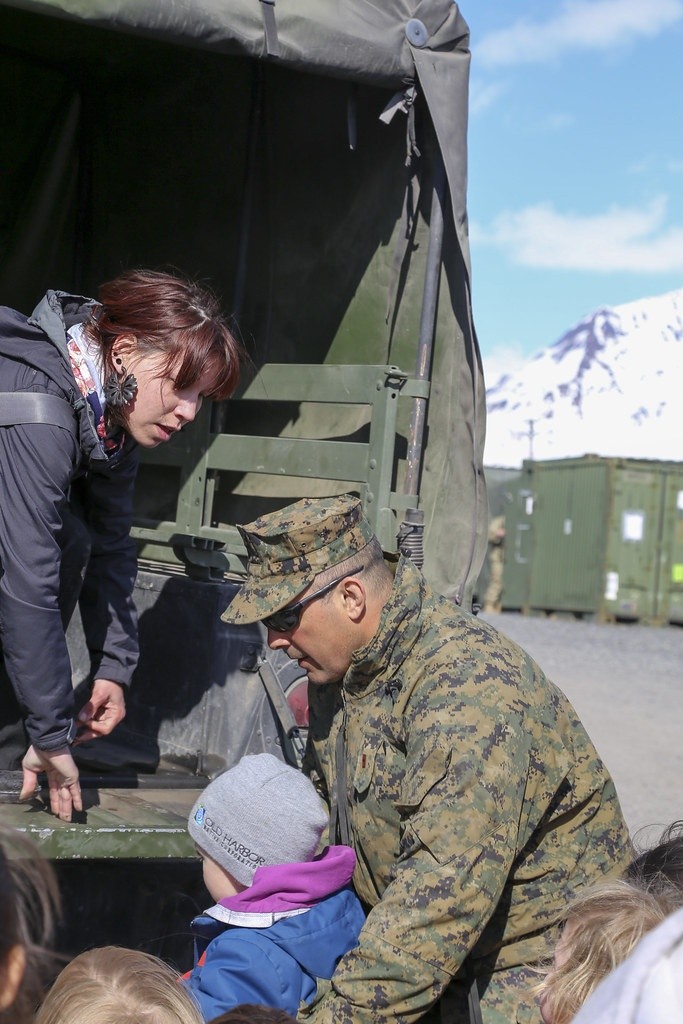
[187, 751, 331, 888]
[220, 494, 374, 625]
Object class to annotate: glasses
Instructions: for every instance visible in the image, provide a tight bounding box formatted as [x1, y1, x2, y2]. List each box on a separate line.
[260, 565, 365, 633]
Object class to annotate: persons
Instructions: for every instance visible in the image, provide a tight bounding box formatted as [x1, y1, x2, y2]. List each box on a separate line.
[33, 947, 204, 1024]
[484, 515, 505, 614]
[171, 752, 366, 1024]
[221, 495, 636, 1024]
[0, 825, 61, 1024]
[509, 820, 683, 1024]
[0, 273, 240, 822]
